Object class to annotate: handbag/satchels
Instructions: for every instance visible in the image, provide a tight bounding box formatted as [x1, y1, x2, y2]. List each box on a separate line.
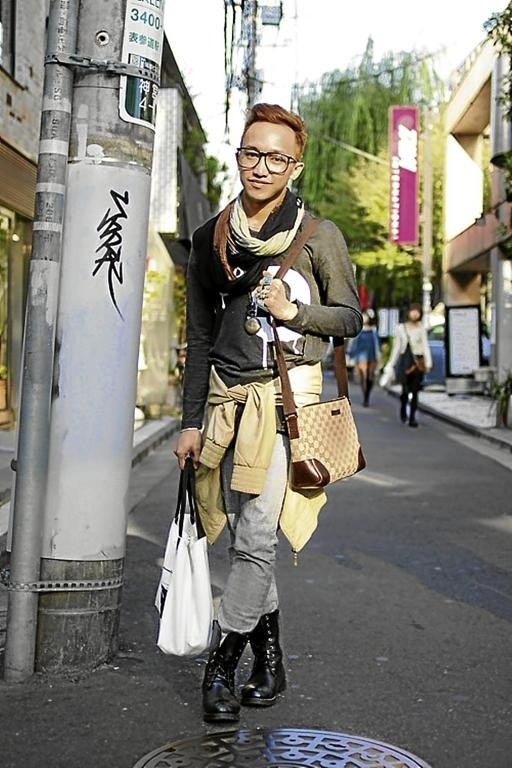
[412, 353, 425, 372]
[282, 395, 368, 492]
[154, 453, 217, 660]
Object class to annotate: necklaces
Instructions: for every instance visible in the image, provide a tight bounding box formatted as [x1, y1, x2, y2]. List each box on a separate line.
[244, 290, 263, 336]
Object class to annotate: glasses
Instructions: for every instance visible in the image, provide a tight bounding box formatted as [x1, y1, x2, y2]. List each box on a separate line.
[236, 146, 298, 175]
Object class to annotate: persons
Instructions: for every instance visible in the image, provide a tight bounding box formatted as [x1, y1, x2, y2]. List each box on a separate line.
[381, 302, 434, 427]
[170, 100, 365, 727]
[344, 307, 383, 408]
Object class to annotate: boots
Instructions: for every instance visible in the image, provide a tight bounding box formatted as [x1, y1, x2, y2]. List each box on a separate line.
[200, 618, 251, 724]
[239, 608, 290, 708]
